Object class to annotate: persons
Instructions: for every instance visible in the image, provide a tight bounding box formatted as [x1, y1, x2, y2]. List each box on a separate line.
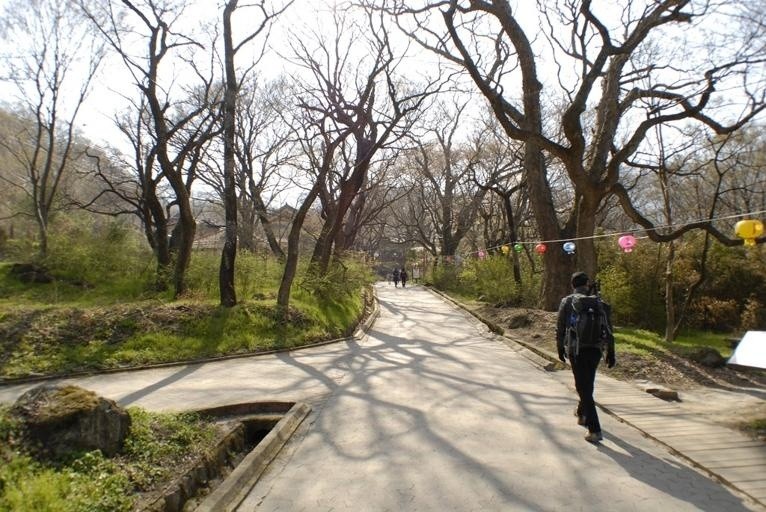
[387, 271, 392, 285]
[555, 270, 616, 444]
[400, 268, 407, 288]
[393, 267, 400, 287]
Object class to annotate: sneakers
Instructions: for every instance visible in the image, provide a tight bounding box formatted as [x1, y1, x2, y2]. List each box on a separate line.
[574, 407, 586, 425]
[585, 432, 602, 442]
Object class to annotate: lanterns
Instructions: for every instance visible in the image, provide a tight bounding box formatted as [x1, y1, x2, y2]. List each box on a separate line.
[734, 217, 765, 249]
[535, 243, 547, 255]
[501, 246, 510, 254]
[617, 234, 638, 255]
[478, 251, 485, 260]
[563, 242, 576, 255]
[514, 244, 523, 254]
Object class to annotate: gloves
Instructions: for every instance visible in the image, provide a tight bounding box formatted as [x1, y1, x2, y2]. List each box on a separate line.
[558, 348, 566, 363]
[605, 354, 616, 368]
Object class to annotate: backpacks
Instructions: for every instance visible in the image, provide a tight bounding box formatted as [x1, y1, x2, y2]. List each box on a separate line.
[568, 293, 611, 348]
